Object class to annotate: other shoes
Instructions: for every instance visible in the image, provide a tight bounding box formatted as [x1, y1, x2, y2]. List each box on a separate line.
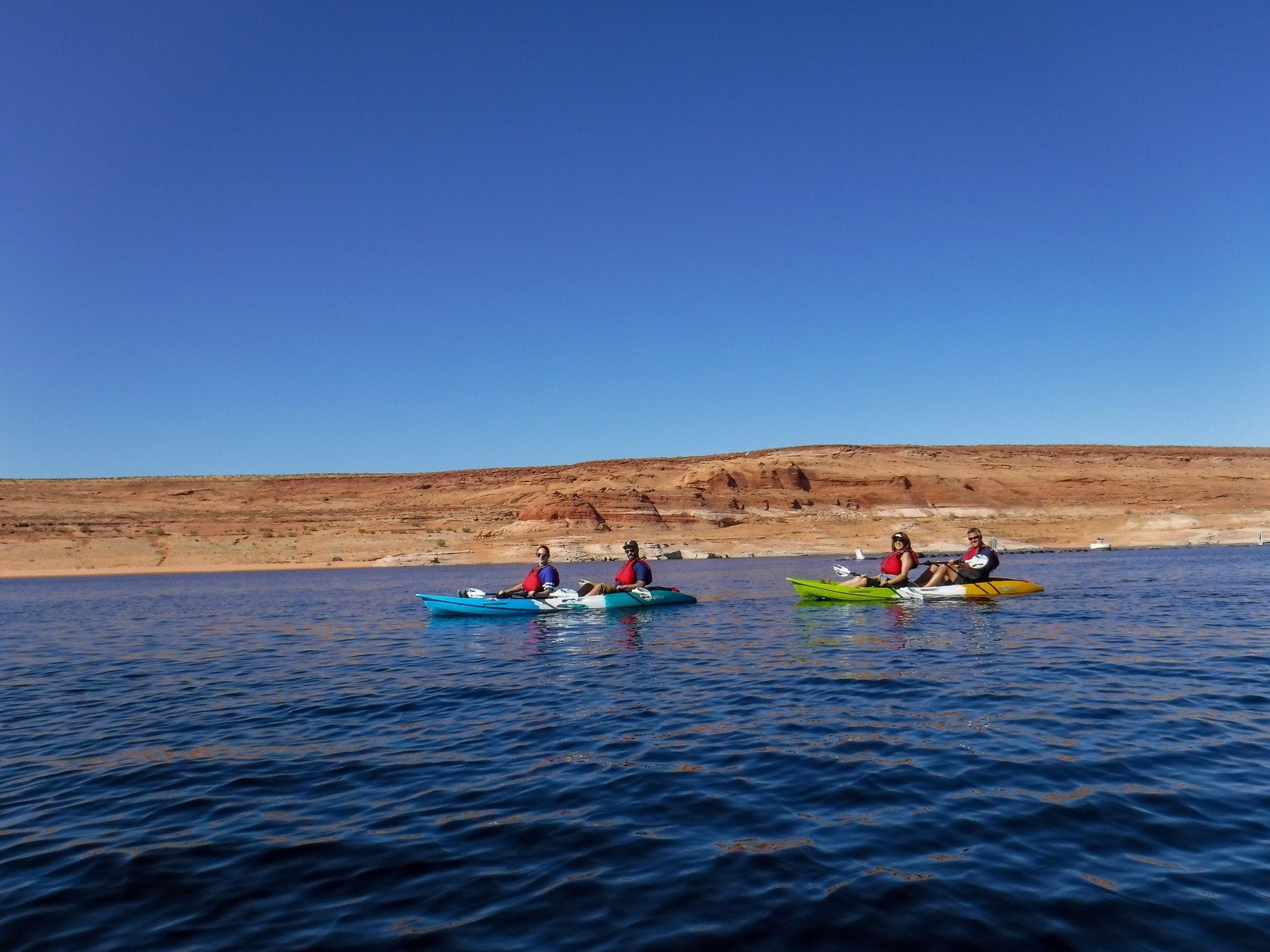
[820, 578, 831, 584]
[456, 589, 469, 597]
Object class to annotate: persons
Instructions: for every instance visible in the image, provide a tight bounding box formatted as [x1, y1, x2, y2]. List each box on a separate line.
[820, 532, 914, 587]
[485, 546, 555, 599]
[914, 528, 993, 588]
[580, 539, 647, 597]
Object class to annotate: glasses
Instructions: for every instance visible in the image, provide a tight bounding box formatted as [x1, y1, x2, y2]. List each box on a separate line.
[536, 553, 549, 557]
[968, 536, 979, 541]
[625, 549, 635, 553]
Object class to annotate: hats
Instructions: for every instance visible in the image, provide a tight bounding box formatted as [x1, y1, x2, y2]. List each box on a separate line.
[892, 533, 905, 542]
[623, 539, 638, 549]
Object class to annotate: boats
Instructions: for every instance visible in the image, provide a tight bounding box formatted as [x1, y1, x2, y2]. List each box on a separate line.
[784, 575, 1045, 603]
[415, 585, 699, 616]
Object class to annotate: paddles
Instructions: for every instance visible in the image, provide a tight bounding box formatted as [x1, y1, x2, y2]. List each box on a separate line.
[465, 587, 578, 604]
[578, 579, 652, 601]
[919, 554, 988, 569]
[833, 563, 923, 600]
